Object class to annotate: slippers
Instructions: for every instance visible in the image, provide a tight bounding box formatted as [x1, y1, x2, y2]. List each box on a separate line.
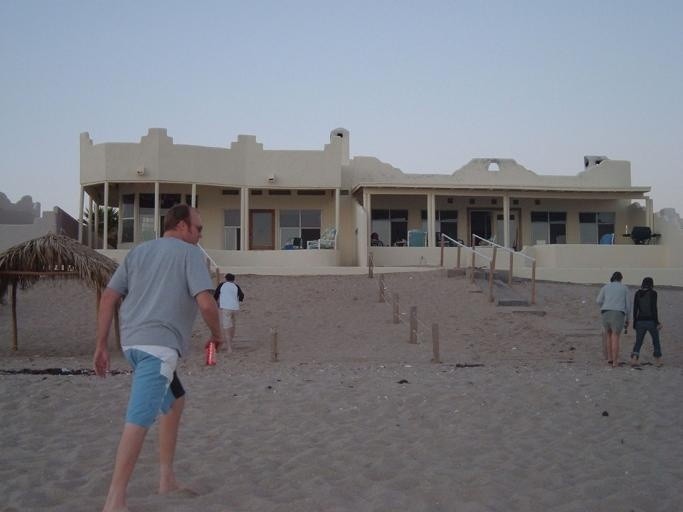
[607, 358, 623, 370]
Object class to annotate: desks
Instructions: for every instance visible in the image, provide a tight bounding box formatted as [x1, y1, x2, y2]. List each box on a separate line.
[621, 224, 663, 245]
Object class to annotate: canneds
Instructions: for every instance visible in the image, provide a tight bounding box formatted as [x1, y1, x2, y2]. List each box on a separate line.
[205, 340, 217, 365]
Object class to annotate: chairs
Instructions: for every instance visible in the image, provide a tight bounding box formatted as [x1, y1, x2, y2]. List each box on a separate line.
[598, 231, 617, 245]
[304, 225, 338, 251]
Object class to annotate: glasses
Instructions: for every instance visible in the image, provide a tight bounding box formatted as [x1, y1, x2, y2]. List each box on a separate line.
[189, 221, 204, 232]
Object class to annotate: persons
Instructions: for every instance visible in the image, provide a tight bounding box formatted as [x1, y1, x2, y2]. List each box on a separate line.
[628, 276, 664, 369]
[211, 272, 245, 353]
[594, 271, 631, 370]
[91, 205, 227, 512]
